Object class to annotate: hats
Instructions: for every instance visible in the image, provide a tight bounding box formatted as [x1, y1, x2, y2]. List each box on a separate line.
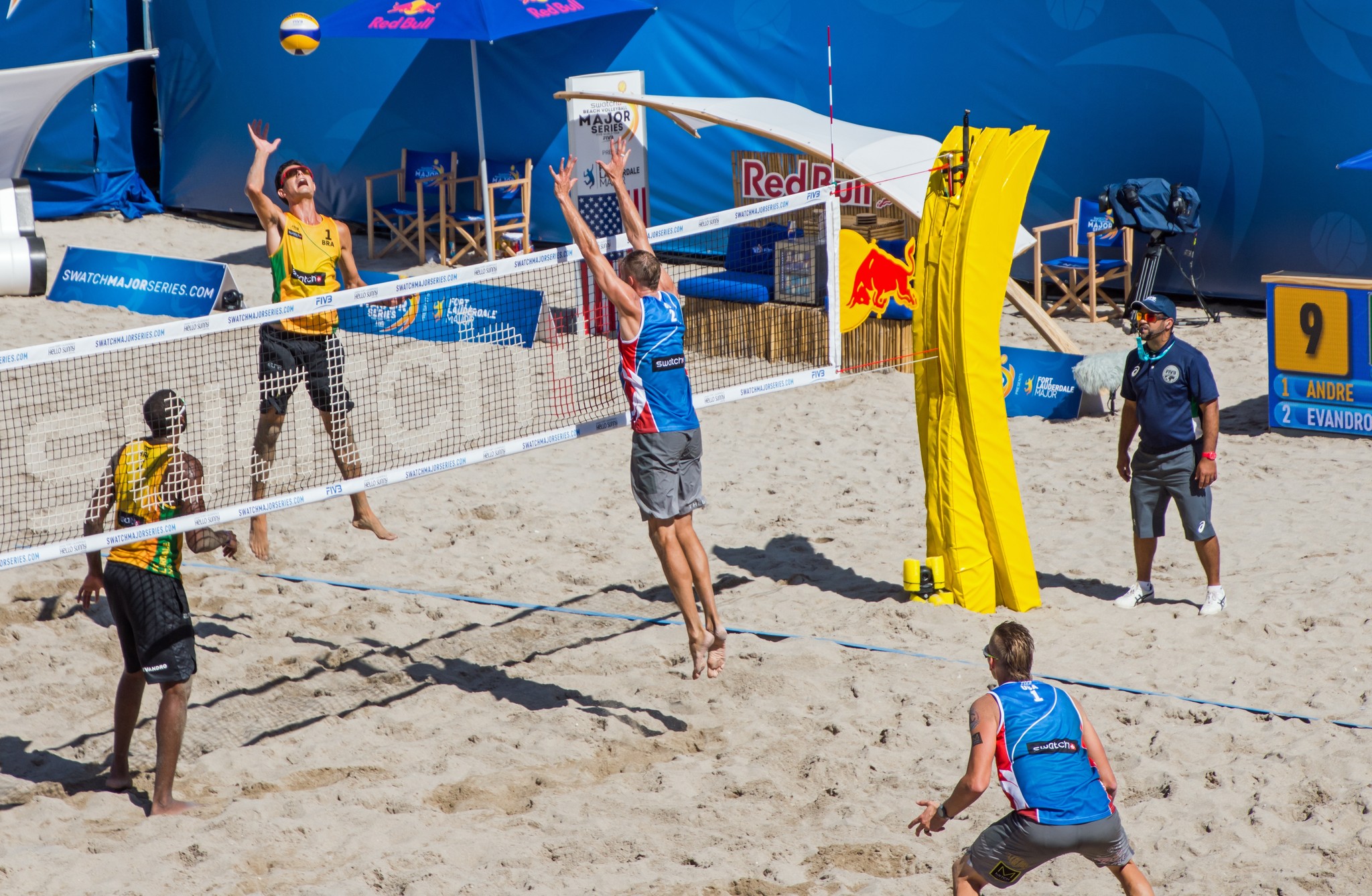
[1131, 294, 1177, 322]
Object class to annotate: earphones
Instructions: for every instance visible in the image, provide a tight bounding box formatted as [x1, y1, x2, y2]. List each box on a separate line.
[1166, 322, 1170, 326]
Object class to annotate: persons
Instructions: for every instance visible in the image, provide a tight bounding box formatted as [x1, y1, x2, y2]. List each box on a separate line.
[242, 120, 396, 562]
[1115, 294, 1227, 614]
[77, 390, 243, 817]
[549, 135, 726, 678]
[909, 621, 1156, 896]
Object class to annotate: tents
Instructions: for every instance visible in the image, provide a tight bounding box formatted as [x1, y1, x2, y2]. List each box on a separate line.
[0, 0, 1372, 304]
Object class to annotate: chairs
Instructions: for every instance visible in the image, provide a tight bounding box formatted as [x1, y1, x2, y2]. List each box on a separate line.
[437, 155, 536, 267]
[823, 234, 917, 321]
[676, 221, 804, 305]
[1030, 196, 1136, 323]
[362, 147, 459, 266]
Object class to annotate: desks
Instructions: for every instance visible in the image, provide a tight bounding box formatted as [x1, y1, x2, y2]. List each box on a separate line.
[1260, 268, 1372, 440]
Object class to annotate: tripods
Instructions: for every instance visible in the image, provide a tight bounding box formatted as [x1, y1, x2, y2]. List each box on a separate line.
[1120, 230, 1220, 335]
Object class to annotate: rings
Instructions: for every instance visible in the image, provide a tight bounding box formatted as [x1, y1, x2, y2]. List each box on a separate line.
[620, 154, 625, 157]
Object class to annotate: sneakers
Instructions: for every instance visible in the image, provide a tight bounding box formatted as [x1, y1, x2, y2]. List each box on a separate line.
[1198, 587, 1227, 617]
[1112, 582, 1156, 609]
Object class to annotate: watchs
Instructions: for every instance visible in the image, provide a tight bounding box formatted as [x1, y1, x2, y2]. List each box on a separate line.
[938, 805, 954, 820]
[1202, 451, 1217, 460]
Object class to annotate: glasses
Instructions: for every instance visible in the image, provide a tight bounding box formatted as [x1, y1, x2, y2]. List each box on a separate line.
[1136, 312, 1168, 323]
[983, 644, 999, 660]
[280, 166, 314, 189]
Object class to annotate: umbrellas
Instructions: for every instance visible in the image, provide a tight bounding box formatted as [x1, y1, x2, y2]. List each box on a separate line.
[324, 0, 658, 263]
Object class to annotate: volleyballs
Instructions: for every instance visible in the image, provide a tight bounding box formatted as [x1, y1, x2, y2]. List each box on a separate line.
[279, 11, 321, 56]
